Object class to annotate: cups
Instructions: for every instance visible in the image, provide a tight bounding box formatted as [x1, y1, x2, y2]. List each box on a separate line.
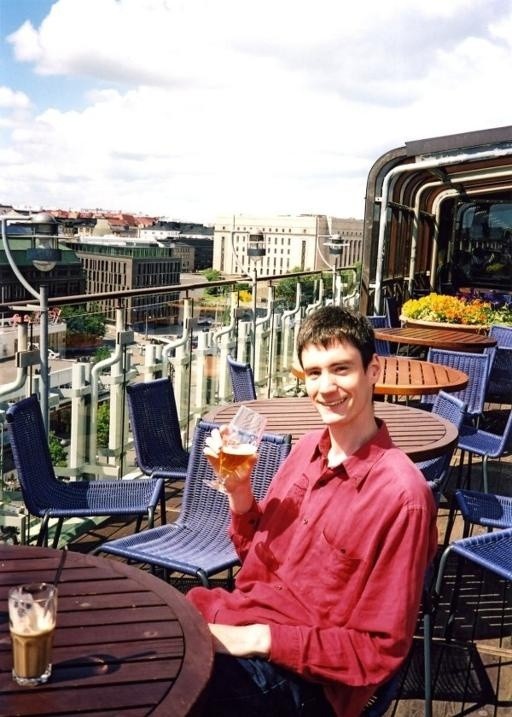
[7, 581, 56, 686]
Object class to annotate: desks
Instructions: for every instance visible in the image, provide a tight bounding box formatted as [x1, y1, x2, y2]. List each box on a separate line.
[1, 545, 215, 716]
[369, 327, 498, 355]
[286, 353, 470, 396]
[199, 397, 459, 464]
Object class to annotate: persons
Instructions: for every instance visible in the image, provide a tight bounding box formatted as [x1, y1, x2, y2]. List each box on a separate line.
[185, 305, 439, 717]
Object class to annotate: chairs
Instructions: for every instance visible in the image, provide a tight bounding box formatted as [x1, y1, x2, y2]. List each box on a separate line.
[123, 377, 193, 533]
[367, 316, 512, 403]
[4, 391, 168, 549]
[440, 489, 512, 546]
[411, 346, 489, 432]
[303, 557, 438, 717]
[449, 405, 512, 494]
[408, 389, 469, 506]
[93, 416, 293, 590]
[434, 528, 512, 673]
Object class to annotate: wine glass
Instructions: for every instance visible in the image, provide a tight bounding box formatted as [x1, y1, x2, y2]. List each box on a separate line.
[202, 404, 267, 496]
